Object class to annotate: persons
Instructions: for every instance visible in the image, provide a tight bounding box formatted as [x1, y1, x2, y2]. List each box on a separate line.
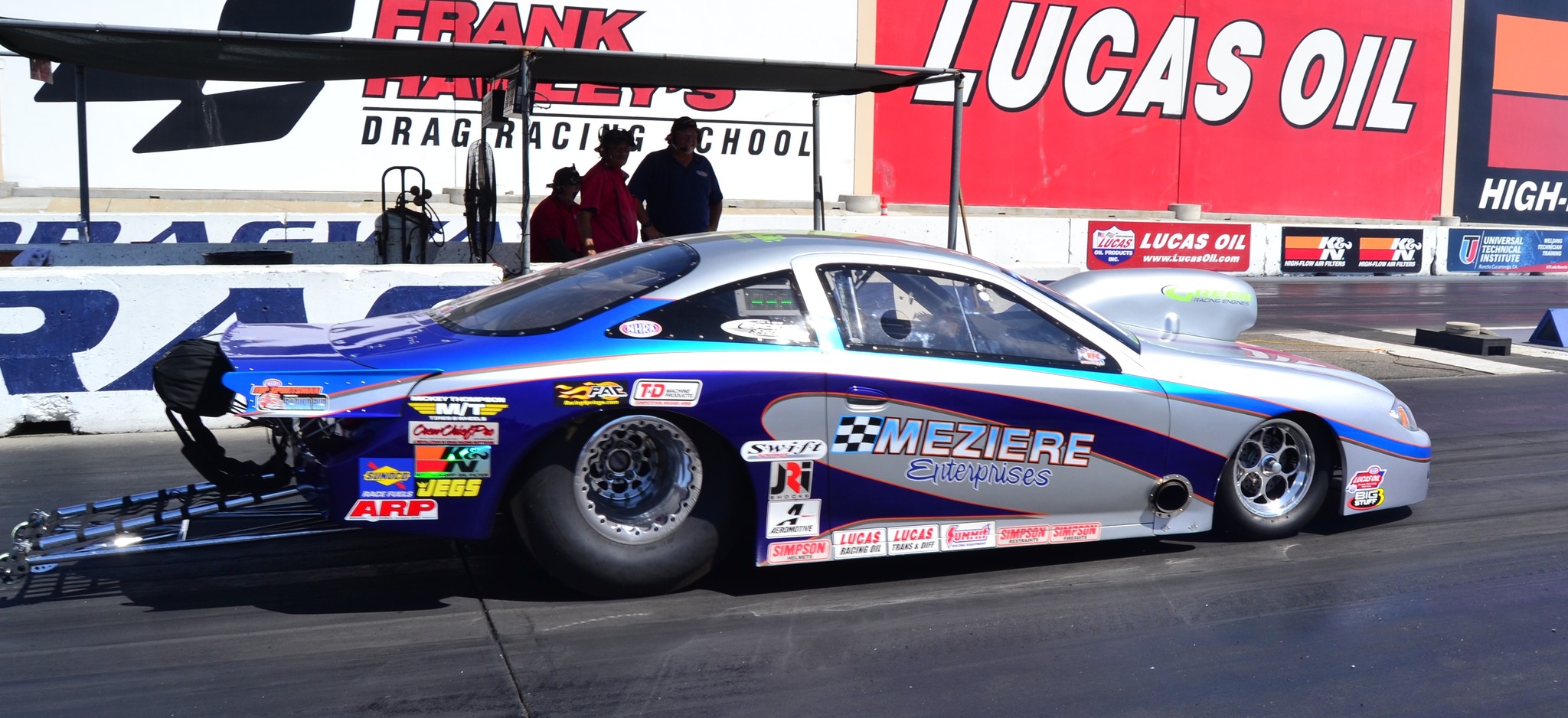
[531, 166, 583, 263]
[577, 129, 637, 257]
[628, 117, 724, 242]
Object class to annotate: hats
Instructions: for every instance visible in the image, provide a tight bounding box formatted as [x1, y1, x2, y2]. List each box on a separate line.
[594, 129, 636, 152]
[546, 164, 581, 188]
[665, 117, 702, 144]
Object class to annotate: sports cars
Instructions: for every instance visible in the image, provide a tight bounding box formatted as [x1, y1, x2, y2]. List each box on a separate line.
[153, 232, 1436, 597]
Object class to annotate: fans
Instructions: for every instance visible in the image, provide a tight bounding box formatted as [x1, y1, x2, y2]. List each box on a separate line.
[463, 138, 497, 263]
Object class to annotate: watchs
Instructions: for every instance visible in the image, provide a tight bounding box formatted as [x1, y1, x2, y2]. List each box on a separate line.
[643, 220, 654, 228]
